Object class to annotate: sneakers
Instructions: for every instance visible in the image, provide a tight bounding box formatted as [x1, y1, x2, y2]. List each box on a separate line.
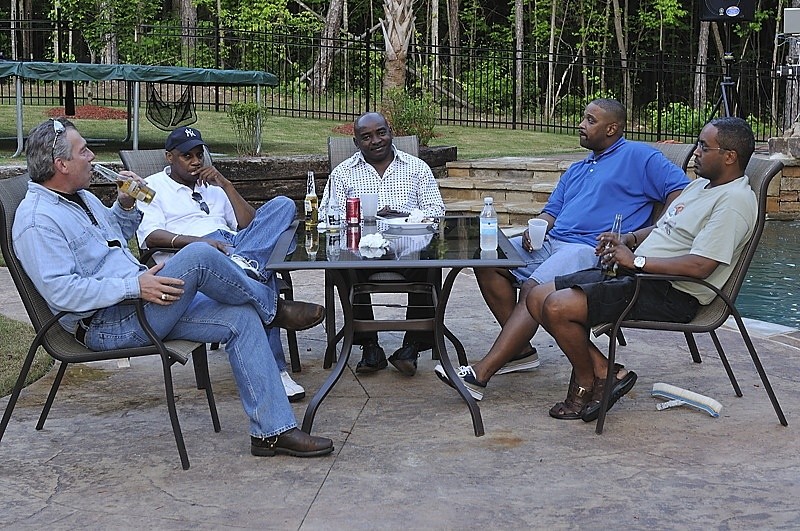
[494, 347, 540, 375]
[434, 366, 487, 400]
[355, 342, 388, 372]
[280, 371, 306, 401]
[228, 254, 260, 278]
[386, 345, 421, 376]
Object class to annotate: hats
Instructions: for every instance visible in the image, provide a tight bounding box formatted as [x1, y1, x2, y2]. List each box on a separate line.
[165, 127, 209, 155]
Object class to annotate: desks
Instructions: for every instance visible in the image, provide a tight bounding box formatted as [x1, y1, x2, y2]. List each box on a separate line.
[266, 218, 528, 434]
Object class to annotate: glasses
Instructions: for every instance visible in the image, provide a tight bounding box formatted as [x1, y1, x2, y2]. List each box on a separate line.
[49, 117, 65, 164]
[697, 142, 735, 156]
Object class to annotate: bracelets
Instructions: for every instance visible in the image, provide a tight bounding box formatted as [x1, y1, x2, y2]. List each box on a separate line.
[171, 234, 181, 248]
[628, 232, 638, 247]
[117, 196, 136, 211]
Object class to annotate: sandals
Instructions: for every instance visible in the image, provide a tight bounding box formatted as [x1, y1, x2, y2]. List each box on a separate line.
[549, 368, 595, 419]
[582, 363, 638, 422]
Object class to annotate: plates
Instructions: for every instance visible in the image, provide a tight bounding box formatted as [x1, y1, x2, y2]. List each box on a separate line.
[381, 216, 435, 229]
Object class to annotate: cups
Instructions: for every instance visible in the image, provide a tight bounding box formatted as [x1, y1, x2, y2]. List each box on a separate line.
[528, 218, 548, 250]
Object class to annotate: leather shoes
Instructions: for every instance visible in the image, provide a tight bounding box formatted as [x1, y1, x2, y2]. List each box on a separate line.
[263, 297, 326, 331]
[251, 426, 334, 456]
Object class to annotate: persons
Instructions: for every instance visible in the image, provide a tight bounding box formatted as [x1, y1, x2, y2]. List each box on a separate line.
[12, 117, 336, 457]
[527, 117, 759, 423]
[131, 126, 305, 402]
[435, 98, 693, 400]
[318, 112, 446, 376]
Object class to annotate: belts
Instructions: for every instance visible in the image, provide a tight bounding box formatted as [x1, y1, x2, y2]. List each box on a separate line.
[76, 315, 93, 346]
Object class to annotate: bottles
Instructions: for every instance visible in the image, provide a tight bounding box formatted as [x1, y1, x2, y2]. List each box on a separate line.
[325, 230, 340, 262]
[601, 214, 622, 277]
[325, 175, 340, 233]
[304, 171, 319, 227]
[479, 197, 498, 250]
[93, 164, 156, 204]
[305, 226, 319, 261]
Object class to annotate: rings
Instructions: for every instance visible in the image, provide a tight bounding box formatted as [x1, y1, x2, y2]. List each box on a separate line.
[610, 253, 613, 257]
[161, 293, 167, 301]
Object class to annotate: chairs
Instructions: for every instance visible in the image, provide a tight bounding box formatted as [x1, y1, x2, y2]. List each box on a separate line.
[646, 141, 697, 227]
[592, 143, 789, 435]
[0, 172, 221, 470]
[118, 148, 302, 374]
[326, 135, 441, 363]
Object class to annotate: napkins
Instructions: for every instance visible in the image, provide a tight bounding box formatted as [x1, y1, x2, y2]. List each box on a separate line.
[317, 229, 327, 233]
[410, 208, 425, 223]
[359, 248, 386, 258]
[358, 232, 387, 249]
[316, 222, 328, 229]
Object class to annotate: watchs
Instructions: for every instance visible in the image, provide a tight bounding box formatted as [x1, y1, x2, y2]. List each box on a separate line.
[633, 256, 647, 272]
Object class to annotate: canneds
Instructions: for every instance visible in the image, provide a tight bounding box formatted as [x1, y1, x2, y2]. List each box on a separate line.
[346, 197, 361, 224]
[347, 224, 361, 250]
